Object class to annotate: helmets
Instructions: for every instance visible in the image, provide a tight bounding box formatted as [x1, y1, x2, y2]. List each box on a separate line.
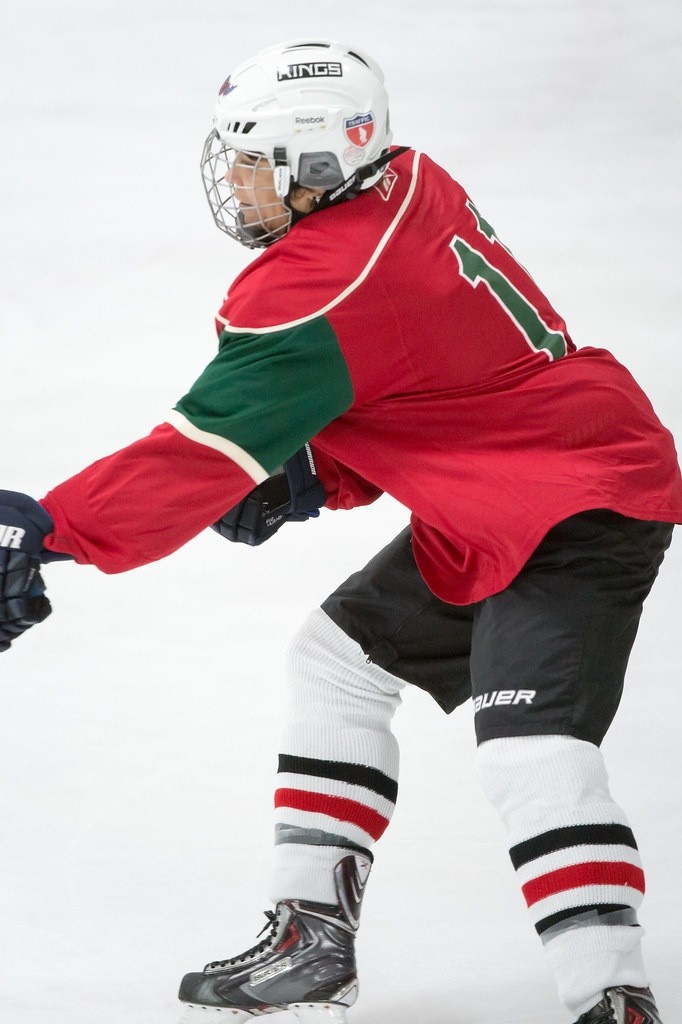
[202, 36, 389, 246]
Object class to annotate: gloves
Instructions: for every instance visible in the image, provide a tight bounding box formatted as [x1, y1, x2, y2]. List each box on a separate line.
[0, 489, 56, 654]
[212, 441, 327, 547]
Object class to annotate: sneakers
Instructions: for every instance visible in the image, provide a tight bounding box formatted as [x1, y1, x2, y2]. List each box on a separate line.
[178, 895, 359, 1023]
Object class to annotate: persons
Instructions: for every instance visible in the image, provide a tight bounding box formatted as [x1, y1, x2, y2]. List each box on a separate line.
[35, 43, 681, 1024]
